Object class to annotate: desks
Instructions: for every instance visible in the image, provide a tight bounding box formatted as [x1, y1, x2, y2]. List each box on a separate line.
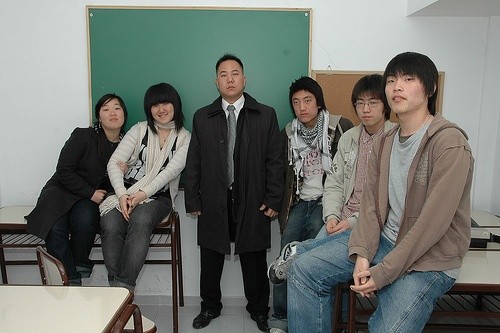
[334, 208, 500, 333]
[0, 204, 184, 333]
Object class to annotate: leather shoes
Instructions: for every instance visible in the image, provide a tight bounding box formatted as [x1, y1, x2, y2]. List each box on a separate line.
[193, 310, 221, 329]
[250, 314, 271, 333]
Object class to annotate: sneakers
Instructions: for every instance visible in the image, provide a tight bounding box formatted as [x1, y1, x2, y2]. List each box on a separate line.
[267, 241, 300, 285]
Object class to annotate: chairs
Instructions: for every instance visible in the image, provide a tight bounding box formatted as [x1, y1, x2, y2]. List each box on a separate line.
[35, 243, 159, 333]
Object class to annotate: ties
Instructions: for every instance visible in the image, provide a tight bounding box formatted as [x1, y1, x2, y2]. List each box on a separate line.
[227, 105, 237, 189]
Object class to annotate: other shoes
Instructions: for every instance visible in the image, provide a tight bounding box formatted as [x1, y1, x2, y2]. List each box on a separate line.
[270, 328, 286, 333]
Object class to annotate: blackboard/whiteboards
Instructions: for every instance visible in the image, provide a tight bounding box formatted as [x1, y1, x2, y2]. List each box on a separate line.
[85, 5, 313, 191]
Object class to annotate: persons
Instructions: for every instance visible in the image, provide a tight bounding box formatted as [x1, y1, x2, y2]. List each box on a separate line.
[285, 53, 476, 333]
[265, 76, 397, 287]
[98, 82, 192, 301]
[186, 53, 284, 332]
[270, 74, 353, 333]
[25, 95, 132, 287]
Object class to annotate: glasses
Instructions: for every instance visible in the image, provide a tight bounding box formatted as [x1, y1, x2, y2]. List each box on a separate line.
[353, 101, 382, 108]
